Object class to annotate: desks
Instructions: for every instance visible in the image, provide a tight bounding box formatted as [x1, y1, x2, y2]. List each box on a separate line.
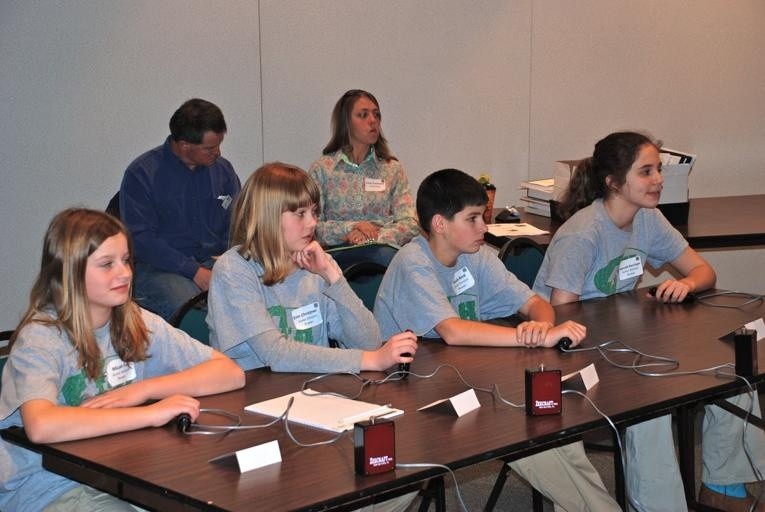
[483, 194, 764, 252]
[0, 284, 764, 511]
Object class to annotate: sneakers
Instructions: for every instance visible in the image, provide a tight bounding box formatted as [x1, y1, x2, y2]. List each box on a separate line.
[699, 483, 765, 512]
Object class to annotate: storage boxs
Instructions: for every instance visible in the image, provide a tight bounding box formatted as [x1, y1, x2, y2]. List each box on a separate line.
[548, 147, 697, 224]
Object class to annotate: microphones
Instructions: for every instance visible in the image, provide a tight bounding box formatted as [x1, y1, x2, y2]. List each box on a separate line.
[399, 329, 413, 377]
[177, 413, 191, 432]
[558, 337, 572, 353]
[649, 287, 695, 304]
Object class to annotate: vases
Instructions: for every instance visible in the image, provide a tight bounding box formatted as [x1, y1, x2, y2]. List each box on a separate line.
[482, 189, 495, 224]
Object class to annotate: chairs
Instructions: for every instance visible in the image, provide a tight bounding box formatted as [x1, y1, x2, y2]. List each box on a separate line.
[497, 238, 545, 290]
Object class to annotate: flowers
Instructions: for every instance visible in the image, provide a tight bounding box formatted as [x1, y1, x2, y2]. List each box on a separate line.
[477, 174, 496, 189]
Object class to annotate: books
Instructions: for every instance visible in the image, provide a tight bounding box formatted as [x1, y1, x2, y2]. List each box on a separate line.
[518, 179, 558, 219]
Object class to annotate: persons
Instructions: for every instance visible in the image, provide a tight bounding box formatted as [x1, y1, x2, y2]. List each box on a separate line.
[0, 205, 247, 510]
[117, 97, 239, 347]
[373, 168, 622, 511]
[530, 131, 765, 510]
[206, 163, 422, 512]
[308, 87, 419, 308]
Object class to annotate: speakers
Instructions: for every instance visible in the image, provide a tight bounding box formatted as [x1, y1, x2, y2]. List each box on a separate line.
[525, 367, 562, 417]
[354, 418, 396, 476]
[734, 329, 758, 378]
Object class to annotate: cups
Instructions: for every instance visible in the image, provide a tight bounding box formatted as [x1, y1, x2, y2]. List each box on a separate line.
[480, 183, 496, 224]
